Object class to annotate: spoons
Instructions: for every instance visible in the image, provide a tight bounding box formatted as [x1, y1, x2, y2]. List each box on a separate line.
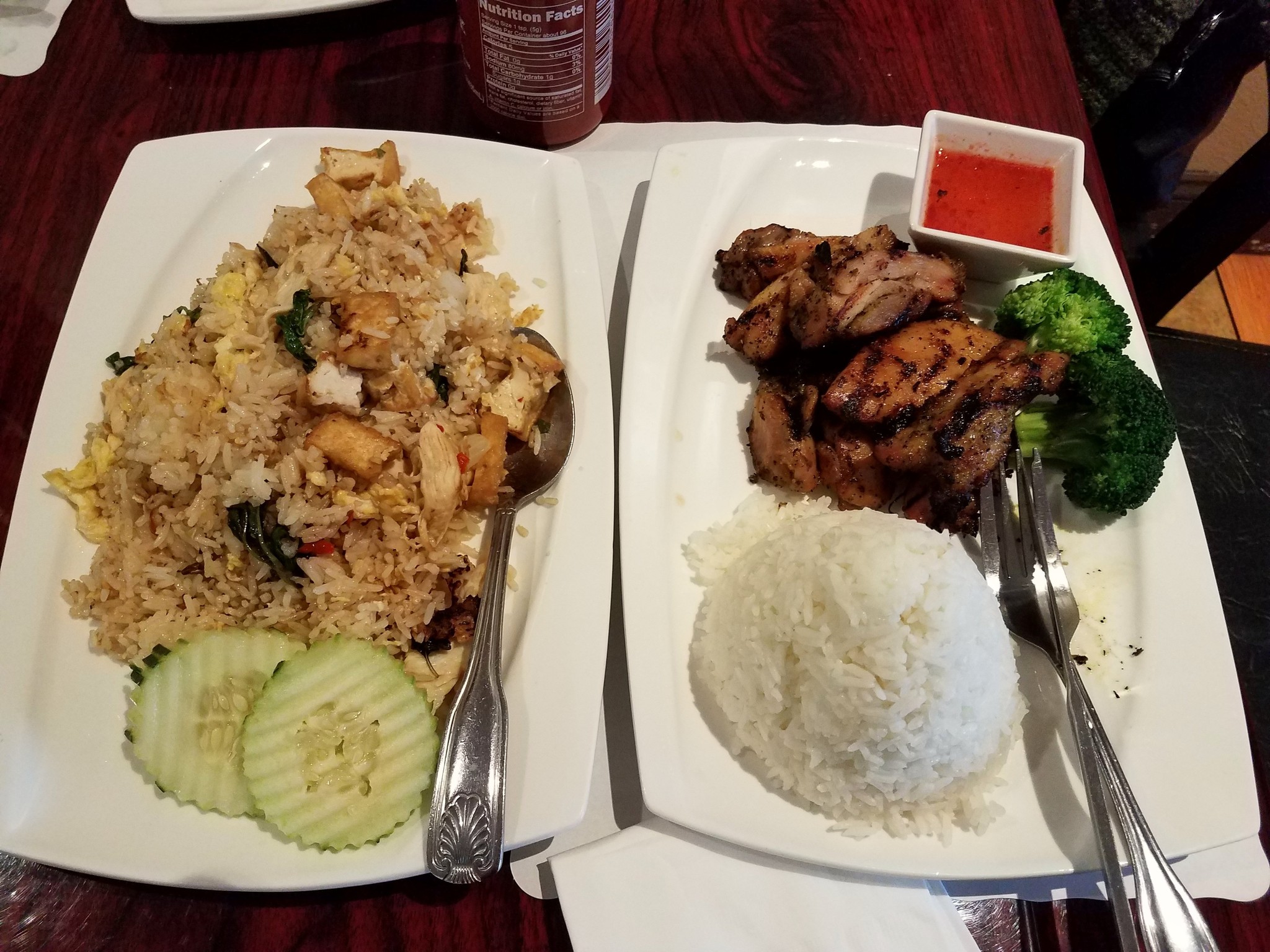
[422, 325, 575, 883]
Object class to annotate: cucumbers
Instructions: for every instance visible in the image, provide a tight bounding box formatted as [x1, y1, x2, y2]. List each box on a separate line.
[120, 628, 443, 849]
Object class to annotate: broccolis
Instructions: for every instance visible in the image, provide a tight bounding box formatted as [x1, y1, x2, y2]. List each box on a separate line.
[990, 266, 1178, 522]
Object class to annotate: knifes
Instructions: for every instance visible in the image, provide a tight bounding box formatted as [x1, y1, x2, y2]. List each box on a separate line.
[1013, 447, 1144, 952]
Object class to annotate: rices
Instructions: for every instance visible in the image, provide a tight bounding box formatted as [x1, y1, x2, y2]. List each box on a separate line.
[680, 489, 1034, 844]
[59, 180, 562, 672]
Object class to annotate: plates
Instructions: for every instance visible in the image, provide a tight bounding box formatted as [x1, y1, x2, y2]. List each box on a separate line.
[2, 125, 617, 893]
[619, 135, 1262, 881]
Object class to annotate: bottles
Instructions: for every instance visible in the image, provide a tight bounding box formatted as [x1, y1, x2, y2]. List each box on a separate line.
[451, 1, 615, 152]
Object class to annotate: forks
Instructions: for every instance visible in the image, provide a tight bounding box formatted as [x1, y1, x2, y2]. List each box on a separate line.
[981, 447, 1219, 951]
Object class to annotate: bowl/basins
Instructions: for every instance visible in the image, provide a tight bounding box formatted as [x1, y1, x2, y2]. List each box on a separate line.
[906, 107, 1088, 276]
